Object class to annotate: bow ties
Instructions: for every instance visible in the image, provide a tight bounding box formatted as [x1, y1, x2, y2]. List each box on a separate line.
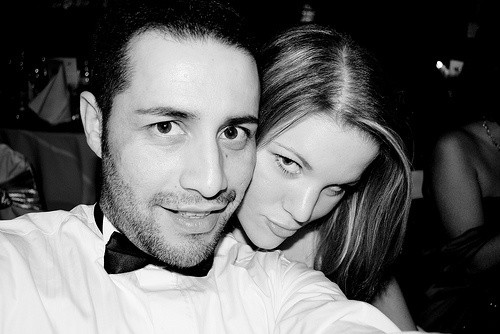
[104, 232, 214, 278]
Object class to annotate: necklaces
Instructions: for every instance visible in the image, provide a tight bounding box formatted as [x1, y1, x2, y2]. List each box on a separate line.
[481, 120, 500, 152]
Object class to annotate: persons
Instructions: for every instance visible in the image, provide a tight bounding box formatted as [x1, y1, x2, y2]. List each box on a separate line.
[431, 112, 500, 334]
[237, 24, 417, 334]
[0, 0, 399, 334]
